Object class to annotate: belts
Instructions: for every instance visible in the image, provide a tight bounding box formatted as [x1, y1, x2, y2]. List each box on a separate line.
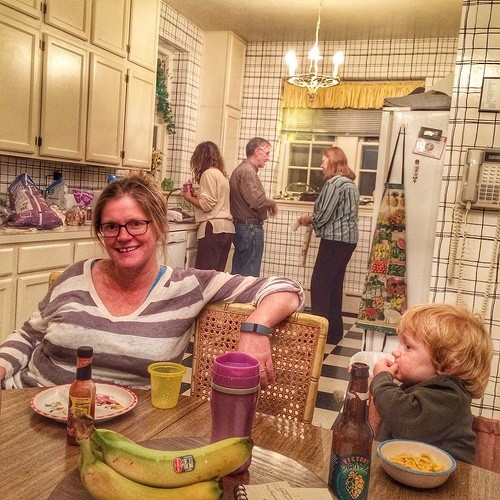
[233, 218, 263, 225]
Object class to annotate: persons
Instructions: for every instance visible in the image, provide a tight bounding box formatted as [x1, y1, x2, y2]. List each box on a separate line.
[298, 147, 359, 344]
[369, 303, 494, 467]
[0, 169, 306, 391]
[229, 138, 278, 276]
[182, 140, 236, 273]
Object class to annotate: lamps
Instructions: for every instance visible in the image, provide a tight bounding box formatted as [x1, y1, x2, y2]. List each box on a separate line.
[284, 1, 347, 102]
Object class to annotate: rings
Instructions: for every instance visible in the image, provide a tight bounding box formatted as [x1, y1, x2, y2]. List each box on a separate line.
[260, 367, 266, 372]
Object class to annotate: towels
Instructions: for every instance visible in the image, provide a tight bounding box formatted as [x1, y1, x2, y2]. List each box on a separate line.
[290, 217, 313, 267]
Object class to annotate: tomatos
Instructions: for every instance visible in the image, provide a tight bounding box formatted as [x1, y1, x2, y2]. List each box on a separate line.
[372, 259, 388, 274]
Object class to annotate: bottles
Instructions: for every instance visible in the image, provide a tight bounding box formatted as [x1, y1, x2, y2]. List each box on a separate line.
[327, 362, 376, 500]
[66, 346, 97, 446]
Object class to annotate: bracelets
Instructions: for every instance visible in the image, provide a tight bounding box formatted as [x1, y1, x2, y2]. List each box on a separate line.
[240, 321, 270, 336]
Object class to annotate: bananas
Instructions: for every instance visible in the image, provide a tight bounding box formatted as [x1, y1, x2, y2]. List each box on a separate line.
[68, 413, 254, 500]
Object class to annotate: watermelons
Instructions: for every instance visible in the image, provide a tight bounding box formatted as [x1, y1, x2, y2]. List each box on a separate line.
[390, 238, 406, 262]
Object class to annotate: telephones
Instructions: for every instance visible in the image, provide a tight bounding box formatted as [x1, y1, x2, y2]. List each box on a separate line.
[459, 147, 499, 211]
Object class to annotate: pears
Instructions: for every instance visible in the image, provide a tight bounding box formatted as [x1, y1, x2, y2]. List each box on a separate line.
[374, 243, 389, 259]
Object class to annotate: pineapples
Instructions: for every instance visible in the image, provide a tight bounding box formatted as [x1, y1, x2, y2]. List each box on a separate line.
[386, 191, 405, 207]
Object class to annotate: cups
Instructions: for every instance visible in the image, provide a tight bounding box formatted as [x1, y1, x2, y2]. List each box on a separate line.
[147, 361, 187, 409]
[210, 352, 262, 475]
[183, 183, 192, 195]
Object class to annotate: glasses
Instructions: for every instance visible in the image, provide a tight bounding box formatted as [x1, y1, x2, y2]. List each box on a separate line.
[95, 220, 155, 238]
[260, 148, 270, 155]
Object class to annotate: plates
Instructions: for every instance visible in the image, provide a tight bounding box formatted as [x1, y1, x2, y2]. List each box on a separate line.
[29, 382, 138, 424]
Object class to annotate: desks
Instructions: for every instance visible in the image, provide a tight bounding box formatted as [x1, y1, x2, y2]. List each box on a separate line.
[0, 387, 499, 500]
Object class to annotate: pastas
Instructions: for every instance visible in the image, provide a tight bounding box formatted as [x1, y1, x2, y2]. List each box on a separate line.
[388, 452, 446, 472]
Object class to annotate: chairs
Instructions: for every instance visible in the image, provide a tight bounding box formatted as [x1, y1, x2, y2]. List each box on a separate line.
[368, 390, 500, 474]
[190, 303, 327, 424]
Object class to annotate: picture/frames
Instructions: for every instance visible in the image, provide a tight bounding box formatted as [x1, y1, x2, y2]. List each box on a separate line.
[477, 77, 500, 112]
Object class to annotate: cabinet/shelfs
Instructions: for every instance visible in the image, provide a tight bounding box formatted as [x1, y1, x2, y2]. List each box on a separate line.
[86, 54, 155, 170]
[90, 0, 162, 72]
[0, 247, 15, 343]
[16, 240, 108, 327]
[0, 0, 89, 42]
[0, 14, 90, 161]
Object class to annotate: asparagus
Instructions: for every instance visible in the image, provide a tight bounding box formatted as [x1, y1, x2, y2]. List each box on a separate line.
[364, 273, 386, 298]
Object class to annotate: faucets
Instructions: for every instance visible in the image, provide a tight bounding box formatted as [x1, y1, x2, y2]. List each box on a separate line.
[166, 187, 181, 202]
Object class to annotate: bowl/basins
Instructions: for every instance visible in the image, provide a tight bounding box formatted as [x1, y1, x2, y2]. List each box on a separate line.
[375, 438, 456, 488]
